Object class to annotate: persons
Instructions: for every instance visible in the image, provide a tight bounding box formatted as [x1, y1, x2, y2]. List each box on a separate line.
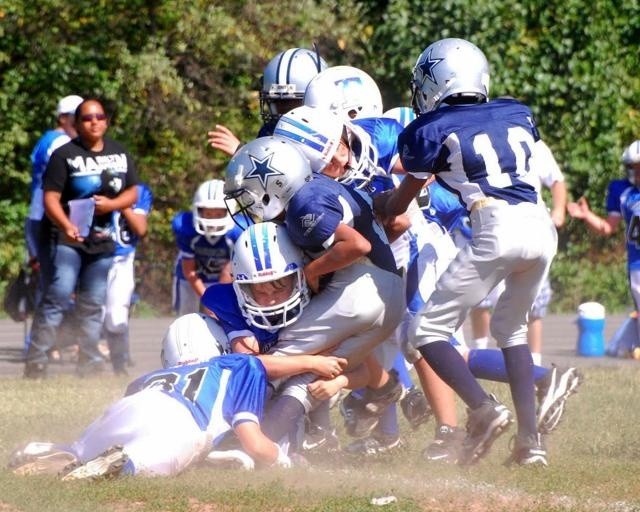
[7, 313, 295, 483]
[471, 95, 565, 395]
[380, 107, 587, 460]
[21, 215, 49, 359]
[374, 37, 558, 466]
[201, 136, 407, 471]
[28, 93, 114, 356]
[207, 48, 330, 157]
[200, 221, 383, 402]
[170, 179, 258, 315]
[24, 99, 134, 378]
[75, 183, 150, 377]
[569, 139, 640, 358]
[274, 110, 479, 460]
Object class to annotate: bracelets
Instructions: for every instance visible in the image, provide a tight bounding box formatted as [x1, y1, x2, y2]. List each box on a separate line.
[271, 444, 291, 470]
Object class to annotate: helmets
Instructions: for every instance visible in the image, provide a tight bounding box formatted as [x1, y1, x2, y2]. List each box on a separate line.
[231, 222, 310, 329]
[259, 47, 328, 133]
[193, 179, 238, 235]
[160, 313, 232, 369]
[57, 95, 84, 116]
[383, 108, 419, 130]
[273, 106, 379, 190]
[224, 136, 313, 230]
[410, 36, 491, 114]
[620, 140, 640, 185]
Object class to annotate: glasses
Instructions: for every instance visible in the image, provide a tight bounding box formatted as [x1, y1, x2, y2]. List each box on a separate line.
[81, 114, 108, 122]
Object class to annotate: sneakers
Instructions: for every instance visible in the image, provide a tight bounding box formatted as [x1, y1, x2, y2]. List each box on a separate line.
[340, 368, 406, 438]
[75, 356, 106, 373]
[206, 440, 254, 474]
[51, 444, 128, 486]
[345, 435, 409, 461]
[25, 364, 46, 375]
[7, 448, 77, 476]
[401, 386, 433, 428]
[300, 423, 342, 454]
[503, 431, 547, 468]
[535, 361, 583, 433]
[422, 423, 467, 461]
[458, 393, 513, 468]
